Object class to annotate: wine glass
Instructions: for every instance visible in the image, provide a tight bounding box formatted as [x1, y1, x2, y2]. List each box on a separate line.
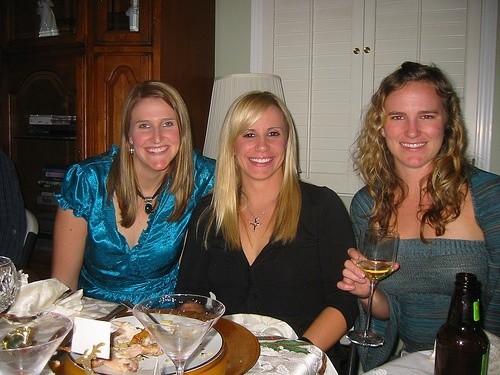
[344, 229, 401, 347]
[134, 293, 226, 375]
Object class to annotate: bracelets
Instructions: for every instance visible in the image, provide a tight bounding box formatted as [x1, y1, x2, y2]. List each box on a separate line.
[298, 336, 314, 345]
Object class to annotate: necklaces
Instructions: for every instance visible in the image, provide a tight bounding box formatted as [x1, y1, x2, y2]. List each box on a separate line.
[240, 197, 278, 232]
[135, 184, 163, 215]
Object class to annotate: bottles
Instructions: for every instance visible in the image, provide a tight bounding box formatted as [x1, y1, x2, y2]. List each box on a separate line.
[434, 272, 492, 375]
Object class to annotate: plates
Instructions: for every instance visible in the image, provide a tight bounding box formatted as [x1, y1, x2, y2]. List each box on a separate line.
[47, 306, 260, 375]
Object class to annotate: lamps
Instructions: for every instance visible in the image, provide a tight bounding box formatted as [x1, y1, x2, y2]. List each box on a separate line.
[203, 72, 286, 161]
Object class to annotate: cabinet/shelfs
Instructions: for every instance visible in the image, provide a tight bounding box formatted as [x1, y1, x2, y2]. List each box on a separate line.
[0, 0, 215, 267]
[250, 0, 499, 198]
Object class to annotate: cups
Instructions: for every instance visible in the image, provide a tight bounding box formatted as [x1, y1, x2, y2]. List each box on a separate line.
[3, 310, 73, 375]
[0, 256, 18, 315]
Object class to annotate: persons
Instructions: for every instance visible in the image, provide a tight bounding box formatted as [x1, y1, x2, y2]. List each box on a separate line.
[336, 62, 500, 373]
[173, 91, 359, 353]
[51, 81, 217, 309]
[0, 144, 27, 265]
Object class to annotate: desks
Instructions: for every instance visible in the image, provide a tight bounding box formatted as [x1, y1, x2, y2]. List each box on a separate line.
[0, 294, 324, 375]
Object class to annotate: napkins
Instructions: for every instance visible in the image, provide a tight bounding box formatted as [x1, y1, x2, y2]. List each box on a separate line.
[7, 278, 83, 316]
